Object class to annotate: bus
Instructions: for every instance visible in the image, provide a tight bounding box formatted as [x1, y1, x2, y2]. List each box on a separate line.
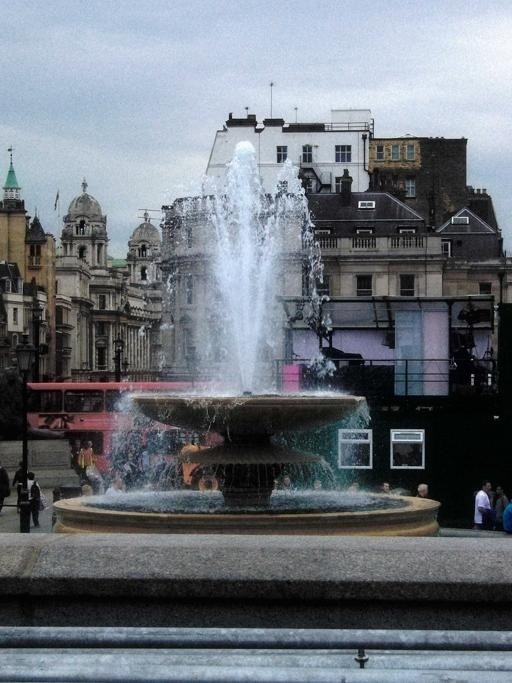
[21, 379, 211, 434]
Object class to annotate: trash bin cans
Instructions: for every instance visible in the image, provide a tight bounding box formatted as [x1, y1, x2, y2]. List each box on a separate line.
[52, 484, 81, 527]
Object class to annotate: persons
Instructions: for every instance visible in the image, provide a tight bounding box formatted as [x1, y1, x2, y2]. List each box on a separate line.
[105, 478, 123, 495]
[0, 461, 11, 517]
[78, 440, 97, 479]
[26, 470, 43, 528]
[347, 486, 357, 493]
[81, 484, 93, 497]
[279, 476, 295, 490]
[70, 438, 86, 479]
[380, 481, 390, 494]
[352, 480, 361, 492]
[12, 460, 24, 514]
[313, 478, 323, 489]
[415, 483, 430, 498]
[470, 476, 512, 534]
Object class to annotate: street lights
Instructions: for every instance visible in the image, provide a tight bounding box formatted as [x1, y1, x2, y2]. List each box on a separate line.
[14, 331, 38, 534]
[122, 357, 130, 379]
[113, 332, 125, 383]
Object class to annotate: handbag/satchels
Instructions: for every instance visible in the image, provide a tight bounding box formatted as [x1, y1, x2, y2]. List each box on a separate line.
[31, 486, 40, 498]
